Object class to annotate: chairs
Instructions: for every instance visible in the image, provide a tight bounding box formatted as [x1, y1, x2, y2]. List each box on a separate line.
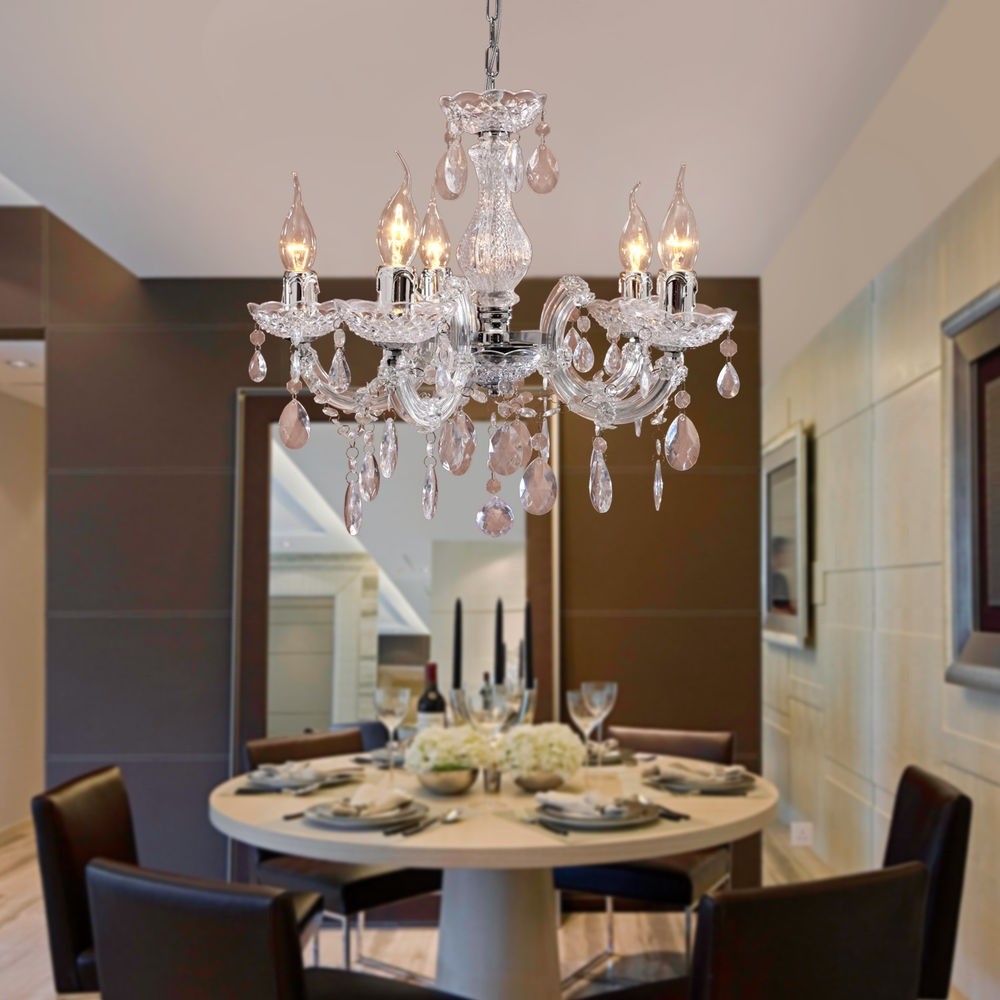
[29, 723, 975, 1000]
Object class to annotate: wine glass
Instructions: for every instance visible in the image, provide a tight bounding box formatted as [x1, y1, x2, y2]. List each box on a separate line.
[374, 685, 411, 788]
[567, 680, 618, 776]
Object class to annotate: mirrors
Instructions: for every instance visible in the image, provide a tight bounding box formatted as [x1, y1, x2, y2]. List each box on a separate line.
[228, 380, 564, 887]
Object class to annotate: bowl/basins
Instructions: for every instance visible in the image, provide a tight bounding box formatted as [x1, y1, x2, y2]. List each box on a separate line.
[415, 767, 478, 794]
[514, 771, 562, 792]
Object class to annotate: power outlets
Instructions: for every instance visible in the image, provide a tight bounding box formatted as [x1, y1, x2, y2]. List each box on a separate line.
[789, 821, 815, 848]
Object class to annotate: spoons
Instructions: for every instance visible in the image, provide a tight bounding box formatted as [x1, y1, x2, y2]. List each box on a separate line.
[235, 783, 320, 795]
[632, 794, 691, 820]
[403, 807, 460, 835]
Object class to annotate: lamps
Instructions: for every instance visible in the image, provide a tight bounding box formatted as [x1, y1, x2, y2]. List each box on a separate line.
[246, 0, 740, 538]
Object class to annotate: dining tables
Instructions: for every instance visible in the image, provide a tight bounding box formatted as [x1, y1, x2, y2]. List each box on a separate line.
[205, 745, 779, 1000]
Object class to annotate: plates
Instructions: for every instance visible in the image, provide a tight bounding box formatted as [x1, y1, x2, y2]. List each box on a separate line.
[534, 798, 659, 828]
[664, 770, 755, 795]
[304, 800, 431, 828]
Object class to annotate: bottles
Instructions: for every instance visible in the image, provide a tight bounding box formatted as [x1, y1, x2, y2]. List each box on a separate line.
[416, 662, 447, 733]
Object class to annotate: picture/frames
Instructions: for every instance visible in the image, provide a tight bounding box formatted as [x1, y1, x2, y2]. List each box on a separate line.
[938, 276, 1000, 695]
[761, 417, 808, 652]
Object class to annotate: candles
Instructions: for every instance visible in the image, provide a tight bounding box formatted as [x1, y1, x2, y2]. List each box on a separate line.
[494, 596, 502, 683]
[452, 597, 462, 691]
[501, 643, 505, 679]
[519, 639, 524, 679]
[525, 600, 534, 691]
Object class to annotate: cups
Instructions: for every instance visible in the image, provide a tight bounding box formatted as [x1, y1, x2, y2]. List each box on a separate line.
[466, 688, 508, 734]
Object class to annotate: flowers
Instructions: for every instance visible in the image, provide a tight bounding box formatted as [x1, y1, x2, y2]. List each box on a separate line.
[502, 721, 592, 781]
[402, 723, 495, 771]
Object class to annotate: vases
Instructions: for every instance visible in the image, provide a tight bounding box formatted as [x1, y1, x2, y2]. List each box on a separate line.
[415, 766, 478, 795]
[510, 764, 567, 794]
